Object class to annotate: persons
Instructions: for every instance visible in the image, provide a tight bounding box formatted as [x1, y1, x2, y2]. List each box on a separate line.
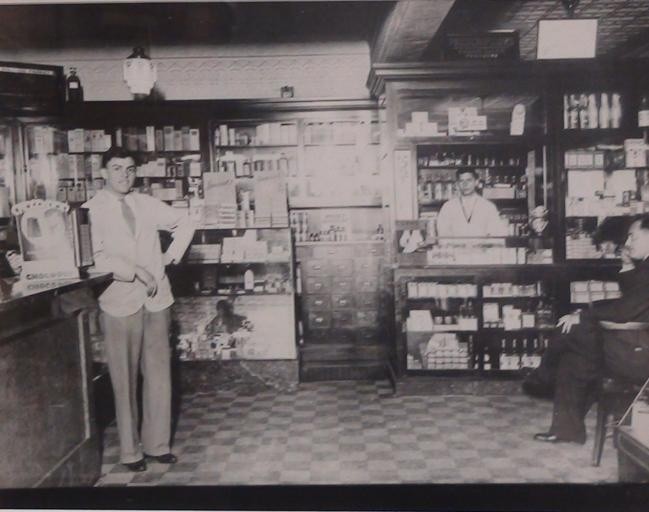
[0, 247, 23, 280]
[434, 164, 508, 245]
[520, 211, 649, 444]
[67, 144, 198, 473]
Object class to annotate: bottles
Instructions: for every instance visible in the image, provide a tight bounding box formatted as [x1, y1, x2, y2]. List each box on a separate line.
[375, 223, 384, 241]
[415, 147, 526, 239]
[122, 45, 159, 100]
[429, 297, 551, 371]
[309, 224, 346, 244]
[243, 269, 255, 294]
[67, 67, 84, 102]
[563, 90, 623, 130]
[215, 147, 291, 180]
[637, 97, 649, 128]
[573, 218, 589, 239]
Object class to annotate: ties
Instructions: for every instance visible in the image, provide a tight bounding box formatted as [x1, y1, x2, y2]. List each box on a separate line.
[119, 198, 136, 237]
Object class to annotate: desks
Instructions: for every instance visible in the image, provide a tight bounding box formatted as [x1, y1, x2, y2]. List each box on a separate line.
[0, 266, 114, 490]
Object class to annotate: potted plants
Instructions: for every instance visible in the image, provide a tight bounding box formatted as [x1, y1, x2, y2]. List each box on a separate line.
[169, 321, 182, 386]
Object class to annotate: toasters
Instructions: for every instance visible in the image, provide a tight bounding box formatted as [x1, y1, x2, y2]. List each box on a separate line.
[138, 177, 151, 195]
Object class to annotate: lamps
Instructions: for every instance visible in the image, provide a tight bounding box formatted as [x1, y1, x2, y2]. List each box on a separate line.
[122, 34, 157, 100]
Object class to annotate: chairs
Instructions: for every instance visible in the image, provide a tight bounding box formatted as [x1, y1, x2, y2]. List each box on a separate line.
[591, 369, 649, 466]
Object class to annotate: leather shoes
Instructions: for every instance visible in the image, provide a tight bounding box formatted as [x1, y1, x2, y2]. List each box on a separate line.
[128, 461, 147, 472]
[534, 432, 572, 445]
[143, 451, 178, 464]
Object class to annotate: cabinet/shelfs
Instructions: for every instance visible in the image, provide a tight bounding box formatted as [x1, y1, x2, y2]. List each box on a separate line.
[294, 243, 392, 345]
[367, 61, 562, 267]
[77, 229, 302, 360]
[543, 60, 649, 267]
[0, 59, 65, 280]
[285, 103, 395, 241]
[60, 100, 290, 227]
[394, 267, 638, 381]
